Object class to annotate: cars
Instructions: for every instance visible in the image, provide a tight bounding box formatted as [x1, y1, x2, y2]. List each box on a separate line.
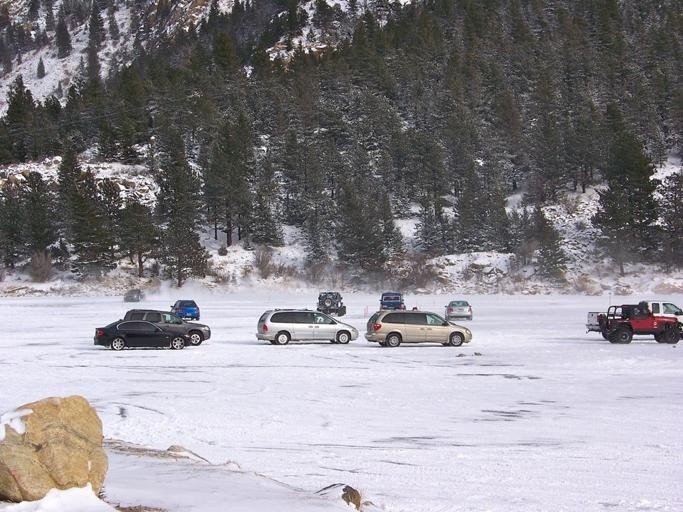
[123, 288, 145, 302]
[444, 300, 473, 321]
[94, 319, 191, 350]
[170, 299, 201, 321]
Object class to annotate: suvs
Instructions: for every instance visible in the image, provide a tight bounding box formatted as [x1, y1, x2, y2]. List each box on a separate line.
[597, 302, 681, 344]
[124, 308, 211, 347]
[317, 291, 347, 317]
[379, 290, 406, 311]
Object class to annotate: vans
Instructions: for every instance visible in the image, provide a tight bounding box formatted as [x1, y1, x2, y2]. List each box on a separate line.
[365, 308, 472, 347]
[255, 308, 359, 345]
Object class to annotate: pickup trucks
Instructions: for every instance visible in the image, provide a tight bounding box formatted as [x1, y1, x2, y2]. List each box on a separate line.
[585, 300, 683, 339]
